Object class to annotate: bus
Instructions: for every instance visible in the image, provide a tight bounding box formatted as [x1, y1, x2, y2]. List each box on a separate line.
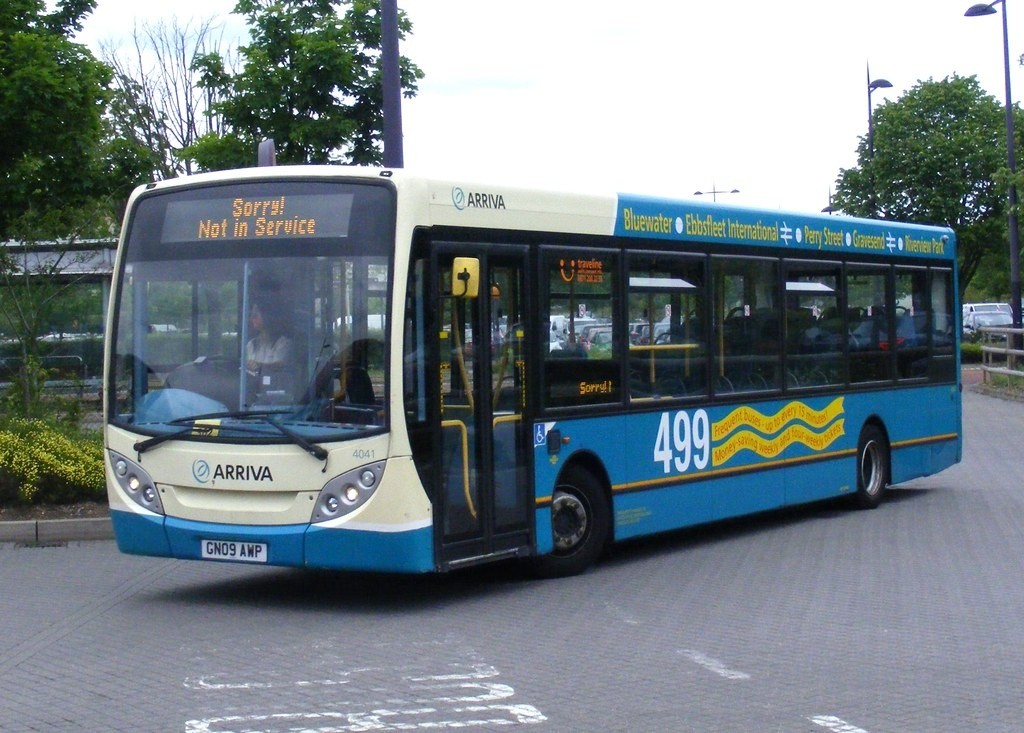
[101, 164, 965, 580]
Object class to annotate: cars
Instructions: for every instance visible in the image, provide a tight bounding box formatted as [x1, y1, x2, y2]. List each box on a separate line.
[966, 303, 1016, 341]
[464, 312, 674, 360]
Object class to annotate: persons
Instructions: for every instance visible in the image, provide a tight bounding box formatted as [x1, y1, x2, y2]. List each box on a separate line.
[247, 302, 293, 392]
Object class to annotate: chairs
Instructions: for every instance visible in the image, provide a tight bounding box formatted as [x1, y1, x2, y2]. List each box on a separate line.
[338, 368, 383, 424]
[630, 299, 913, 398]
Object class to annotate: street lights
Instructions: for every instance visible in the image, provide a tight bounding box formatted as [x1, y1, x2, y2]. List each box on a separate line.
[864, 60, 896, 220]
[964, 1, 1023, 382]
